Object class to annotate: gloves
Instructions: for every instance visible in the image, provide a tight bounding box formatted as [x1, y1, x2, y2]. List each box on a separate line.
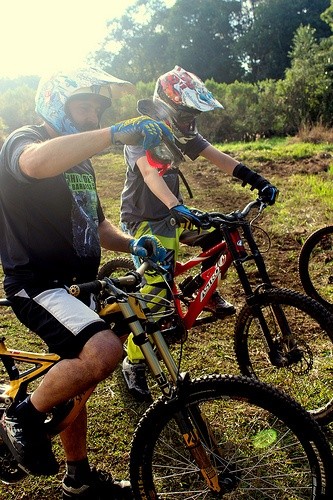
[128, 235, 166, 262]
[172, 204, 210, 230]
[110, 117, 176, 150]
[261, 181, 279, 207]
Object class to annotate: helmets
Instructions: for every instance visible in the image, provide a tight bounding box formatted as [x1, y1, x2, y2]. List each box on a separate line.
[153, 66, 224, 146]
[33, 60, 136, 136]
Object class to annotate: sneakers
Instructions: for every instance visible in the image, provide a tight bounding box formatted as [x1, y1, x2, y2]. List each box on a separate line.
[122, 356, 152, 402]
[178, 275, 235, 317]
[62, 468, 132, 500]
[0, 404, 60, 476]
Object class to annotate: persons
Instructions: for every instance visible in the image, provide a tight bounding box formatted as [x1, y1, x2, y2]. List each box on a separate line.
[0, 67, 176, 500]
[120, 66, 279, 397]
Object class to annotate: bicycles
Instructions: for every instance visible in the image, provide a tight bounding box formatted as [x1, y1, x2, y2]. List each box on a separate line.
[96, 190, 333, 428]
[0, 256, 333, 500]
[298, 225, 333, 314]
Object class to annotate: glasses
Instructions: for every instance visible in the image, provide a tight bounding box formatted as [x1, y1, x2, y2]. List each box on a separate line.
[176, 109, 199, 126]
